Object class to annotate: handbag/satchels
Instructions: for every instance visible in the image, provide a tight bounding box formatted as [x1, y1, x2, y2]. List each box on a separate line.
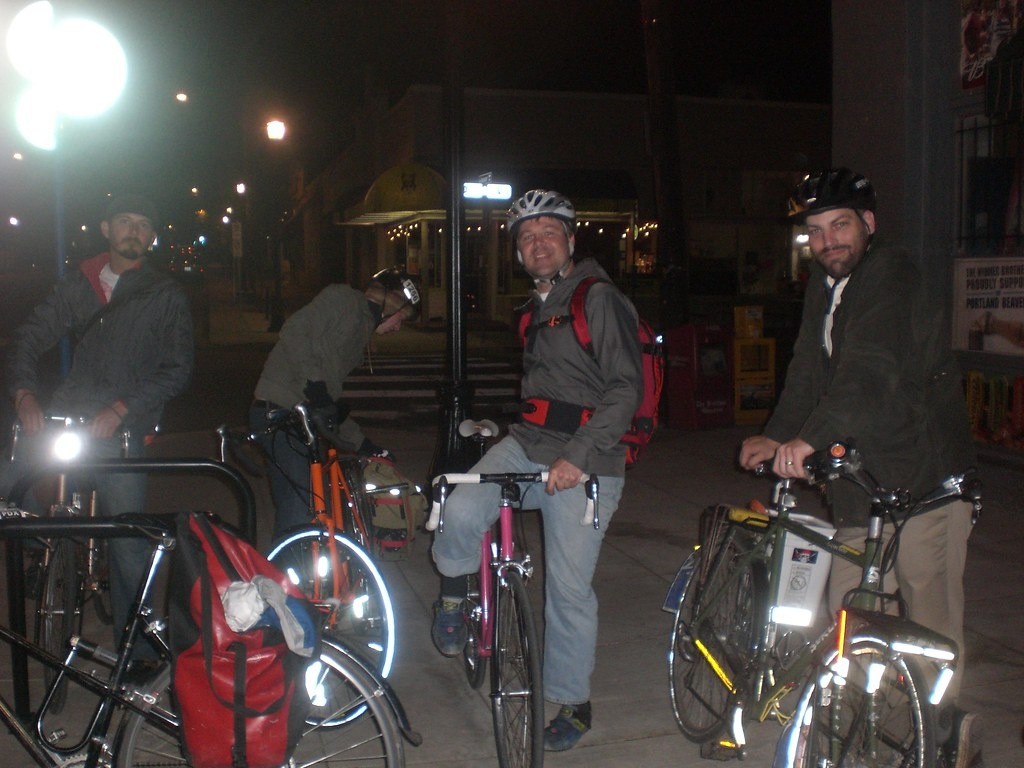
[167, 513, 322, 768]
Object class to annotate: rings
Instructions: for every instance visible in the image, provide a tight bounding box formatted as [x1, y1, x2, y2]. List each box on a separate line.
[786, 462, 793, 465]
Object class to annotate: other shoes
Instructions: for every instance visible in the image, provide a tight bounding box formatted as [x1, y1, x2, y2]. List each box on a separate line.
[936, 708, 982, 768]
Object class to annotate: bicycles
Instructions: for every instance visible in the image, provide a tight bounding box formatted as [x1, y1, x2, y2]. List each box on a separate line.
[423, 470, 598, 768]
[1, 397, 423, 768]
[667, 434, 988, 767]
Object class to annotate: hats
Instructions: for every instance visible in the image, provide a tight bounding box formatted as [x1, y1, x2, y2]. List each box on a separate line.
[106, 194, 156, 225]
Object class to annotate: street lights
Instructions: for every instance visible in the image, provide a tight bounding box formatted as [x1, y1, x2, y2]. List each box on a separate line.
[265, 114, 288, 331]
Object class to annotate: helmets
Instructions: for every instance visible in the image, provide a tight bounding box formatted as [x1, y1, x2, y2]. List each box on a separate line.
[505, 189, 576, 232]
[785, 168, 876, 221]
[371, 263, 422, 321]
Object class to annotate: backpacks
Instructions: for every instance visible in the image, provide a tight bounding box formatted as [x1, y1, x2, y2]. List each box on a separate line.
[360, 459, 427, 562]
[517, 276, 665, 469]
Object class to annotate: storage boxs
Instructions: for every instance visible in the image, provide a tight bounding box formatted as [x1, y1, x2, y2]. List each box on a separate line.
[730, 306, 776, 427]
[663, 327, 735, 429]
[764, 507, 838, 631]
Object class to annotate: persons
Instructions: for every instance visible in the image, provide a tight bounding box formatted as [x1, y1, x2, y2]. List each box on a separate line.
[3, 194, 195, 690]
[739, 169, 981, 768]
[431, 189, 645, 752]
[248, 265, 423, 569]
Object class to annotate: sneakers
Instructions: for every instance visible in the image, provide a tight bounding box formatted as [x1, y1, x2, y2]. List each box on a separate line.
[432, 593, 467, 657]
[543, 703, 591, 752]
